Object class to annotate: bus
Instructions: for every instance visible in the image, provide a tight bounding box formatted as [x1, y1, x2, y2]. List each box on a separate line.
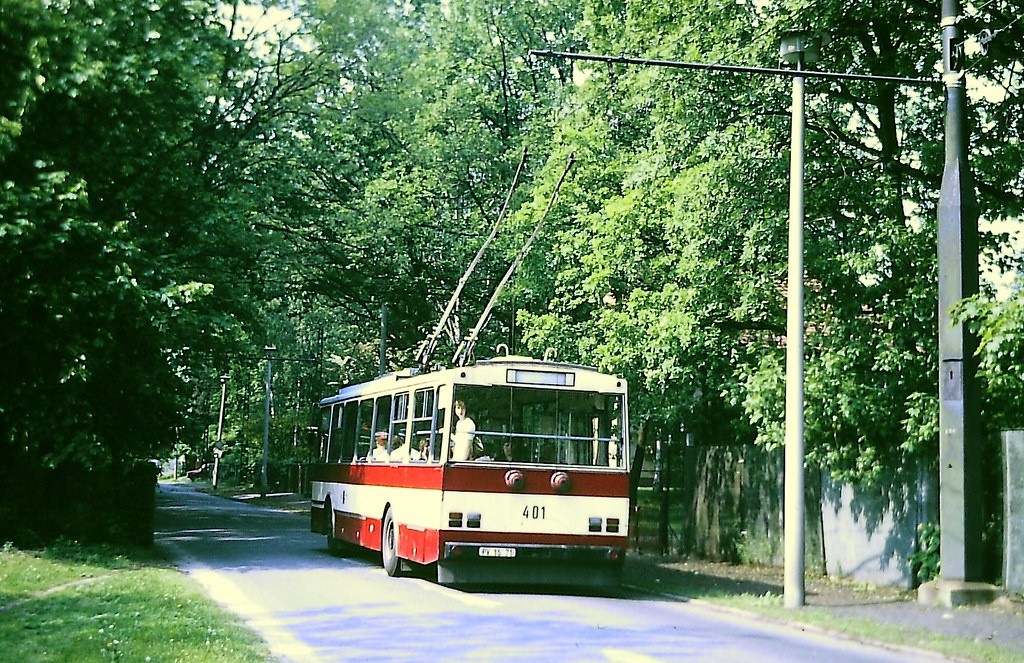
[308, 348, 631, 587]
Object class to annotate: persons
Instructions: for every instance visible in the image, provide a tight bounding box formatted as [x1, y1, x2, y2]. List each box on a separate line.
[361, 400, 531, 463]
